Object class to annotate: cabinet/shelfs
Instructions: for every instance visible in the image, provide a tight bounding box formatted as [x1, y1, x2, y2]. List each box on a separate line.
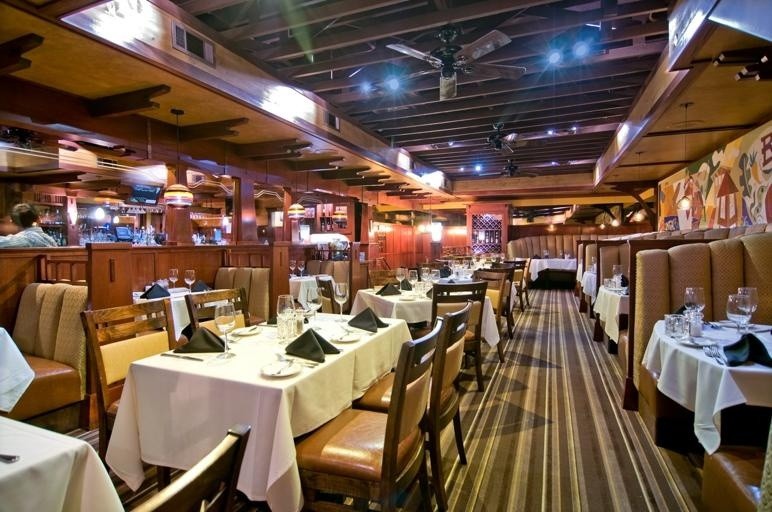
[471, 213, 502, 255]
[32, 193, 65, 245]
[316, 203, 349, 235]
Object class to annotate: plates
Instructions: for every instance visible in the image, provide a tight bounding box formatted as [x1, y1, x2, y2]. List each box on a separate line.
[231, 327, 265, 337]
[331, 332, 361, 343]
[679, 337, 713, 347]
[259, 360, 301, 377]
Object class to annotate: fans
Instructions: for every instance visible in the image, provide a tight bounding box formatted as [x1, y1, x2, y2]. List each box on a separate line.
[474, 123, 527, 154]
[384, 29, 527, 102]
[479, 160, 538, 177]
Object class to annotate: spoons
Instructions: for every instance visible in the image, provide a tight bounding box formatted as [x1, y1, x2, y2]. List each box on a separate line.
[703, 344, 728, 365]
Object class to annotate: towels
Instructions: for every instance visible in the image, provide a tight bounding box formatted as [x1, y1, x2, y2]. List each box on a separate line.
[399, 279, 413, 290]
[140, 284, 169, 299]
[349, 308, 389, 332]
[374, 284, 402, 295]
[285, 329, 339, 363]
[191, 280, 211, 293]
[174, 326, 229, 354]
[674, 303, 698, 315]
[440, 267, 451, 278]
[724, 333, 768, 368]
[621, 275, 630, 287]
[533, 255, 541, 259]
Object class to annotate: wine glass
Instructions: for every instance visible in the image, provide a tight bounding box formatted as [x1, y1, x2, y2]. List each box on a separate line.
[395, 252, 505, 296]
[126, 207, 165, 215]
[592, 256, 597, 265]
[559, 250, 563, 259]
[214, 302, 236, 360]
[169, 268, 195, 293]
[277, 283, 350, 345]
[684, 286, 759, 337]
[613, 265, 624, 278]
[289, 259, 305, 277]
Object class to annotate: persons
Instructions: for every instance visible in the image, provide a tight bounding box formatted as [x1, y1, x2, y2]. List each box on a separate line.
[0, 202, 57, 248]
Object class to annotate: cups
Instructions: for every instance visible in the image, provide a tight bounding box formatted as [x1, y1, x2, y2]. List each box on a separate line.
[603, 278, 616, 290]
[664, 313, 685, 338]
[414, 282, 426, 299]
[543, 249, 549, 259]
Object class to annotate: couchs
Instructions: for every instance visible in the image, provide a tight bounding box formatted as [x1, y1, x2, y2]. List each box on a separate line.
[701, 424, 771, 512]
[600, 223, 772, 285]
[574, 244, 583, 297]
[306, 260, 350, 314]
[506, 234, 633, 260]
[0, 278, 89, 420]
[214, 267, 271, 326]
[633, 233, 771, 443]
[584, 229, 692, 305]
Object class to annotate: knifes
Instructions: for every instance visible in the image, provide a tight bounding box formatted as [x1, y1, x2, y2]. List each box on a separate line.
[160, 353, 203, 361]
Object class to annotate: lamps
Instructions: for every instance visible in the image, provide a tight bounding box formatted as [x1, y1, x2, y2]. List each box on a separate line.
[676, 101, 694, 211]
[164, 109, 194, 210]
[632, 150, 644, 221]
[287, 158, 306, 222]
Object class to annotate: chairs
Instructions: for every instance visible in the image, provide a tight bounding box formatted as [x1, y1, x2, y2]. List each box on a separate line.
[473, 271, 507, 363]
[503, 261, 526, 312]
[515, 258, 531, 306]
[295, 316, 443, 511]
[355, 299, 473, 511]
[79, 297, 177, 492]
[185, 287, 250, 336]
[481, 268, 513, 339]
[129, 423, 252, 512]
[414, 281, 488, 392]
[368, 270, 396, 288]
[491, 263, 515, 326]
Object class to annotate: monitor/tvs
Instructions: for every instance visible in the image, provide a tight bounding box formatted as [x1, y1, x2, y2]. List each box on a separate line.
[123, 183, 163, 207]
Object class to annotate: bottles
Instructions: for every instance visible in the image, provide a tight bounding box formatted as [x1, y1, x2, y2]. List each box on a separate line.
[321, 218, 332, 231]
[473, 230, 500, 244]
[42, 208, 67, 246]
[80, 224, 158, 247]
